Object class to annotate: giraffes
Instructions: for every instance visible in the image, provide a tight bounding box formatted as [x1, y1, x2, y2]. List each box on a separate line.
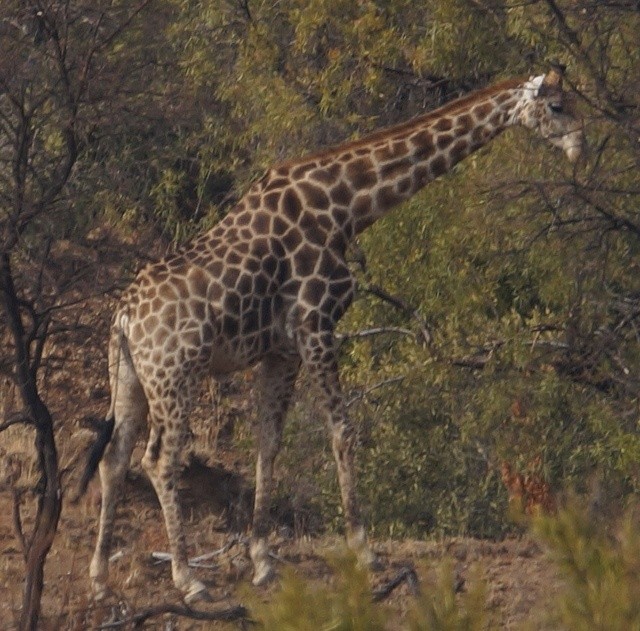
[64, 58, 591, 608]
